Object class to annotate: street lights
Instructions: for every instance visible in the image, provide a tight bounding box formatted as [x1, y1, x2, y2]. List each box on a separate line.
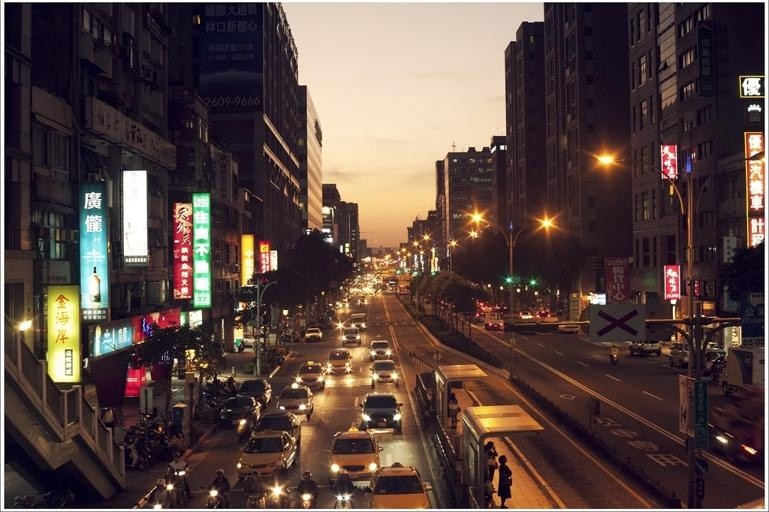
[469, 211, 554, 383]
[595, 150, 764, 500]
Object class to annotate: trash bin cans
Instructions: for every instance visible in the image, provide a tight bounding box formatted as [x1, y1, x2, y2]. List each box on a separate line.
[236, 338, 241, 347]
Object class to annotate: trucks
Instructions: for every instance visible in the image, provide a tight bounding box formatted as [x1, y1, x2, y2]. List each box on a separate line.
[484, 313, 504, 330]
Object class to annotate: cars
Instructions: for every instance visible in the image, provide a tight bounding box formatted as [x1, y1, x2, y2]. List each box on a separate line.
[220, 397, 260, 431]
[558, 323, 580, 335]
[368, 360, 399, 389]
[274, 384, 312, 421]
[629, 342, 662, 356]
[364, 466, 433, 509]
[240, 333, 270, 348]
[324, 430, 388, 489]
[369, 341, 391, 362]
[342, 327, 361, 346]
[234, 428, 296, 486]
[236, 379, 272, 409]
[326, 349, 352, 375]
[475, 300, 556, 319]
[296, 360, 325, 392]
[303, 327, 322, 342]
[358, 393, 403, 435]
[672, 340, 731, 387]
[254, 411, 300, 451]
[325, 274, 398, 329]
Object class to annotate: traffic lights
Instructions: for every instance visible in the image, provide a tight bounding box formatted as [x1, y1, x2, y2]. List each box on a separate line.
[530, 280, 536, 287]
[505, 278, 521, 284]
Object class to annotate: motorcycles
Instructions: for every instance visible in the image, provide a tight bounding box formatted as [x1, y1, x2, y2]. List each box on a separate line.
[123, 409, 187, 465]
[608, 350, 617, 365]
[145, 462, 353, 508]
[198, 375, 238, 422]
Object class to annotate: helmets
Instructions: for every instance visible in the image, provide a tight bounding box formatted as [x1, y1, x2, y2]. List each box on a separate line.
[338, 470, 349, 480]
[216, 468, 225, 473]
[303, 472, 312, 479]
[172, 452, 180, 458]
[155, 478, 166, 486]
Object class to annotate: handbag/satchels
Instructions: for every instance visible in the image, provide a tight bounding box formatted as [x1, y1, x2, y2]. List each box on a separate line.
[508, 477, 512, 486]
[494, 461, 498, 469]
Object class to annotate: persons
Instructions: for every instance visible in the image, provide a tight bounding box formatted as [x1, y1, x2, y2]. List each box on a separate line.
[609, 345, 619, 364]
[155, 424, 166, 451]
[148, 477, 172, 508]
[166, 451, 194, 500]
[241, 470, 269, 509]
[210, 467, 232, 509]
[707, 358, 728, 384]
[8, 481, 75, 508]
[448, 392, 459, 428]
[485, 441, 498, 493]
[498, 454, 512, 508]
[101, 406, 117, 437]
[225, 376, 233, 382]
[295, 471, 319, 509]
[331, 473, 354, 509]
[241, 404, 255, 429]
